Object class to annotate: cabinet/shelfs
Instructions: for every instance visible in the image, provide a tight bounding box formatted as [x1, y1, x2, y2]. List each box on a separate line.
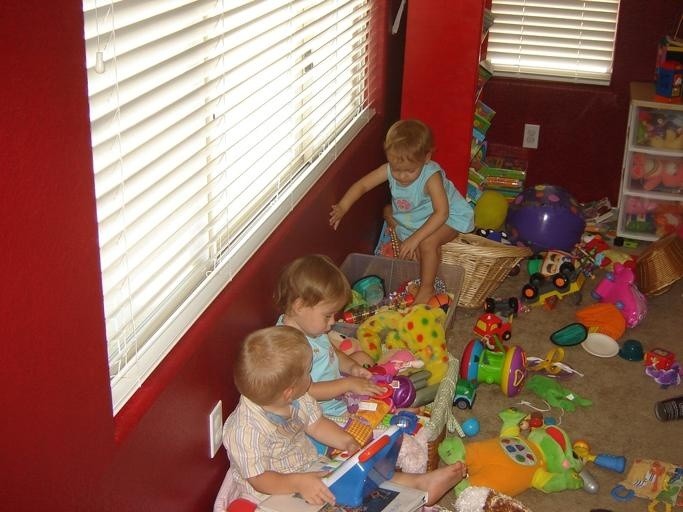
[399, 0, 492, 200]
[612, 81, 682, 247]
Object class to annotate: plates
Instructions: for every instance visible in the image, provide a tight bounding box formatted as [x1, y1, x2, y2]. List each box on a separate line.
[579, 333, 619, 359]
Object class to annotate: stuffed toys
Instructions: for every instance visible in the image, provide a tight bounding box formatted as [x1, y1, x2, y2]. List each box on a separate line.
[584, 236, 640, 273]
[325, 303, 450, 386]
[438, 407, 584, 511]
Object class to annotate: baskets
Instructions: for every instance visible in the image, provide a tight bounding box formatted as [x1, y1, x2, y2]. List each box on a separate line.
[410, 233, 532, 312]
[382, 343, 463, 475]
[637, 230, 683, 297]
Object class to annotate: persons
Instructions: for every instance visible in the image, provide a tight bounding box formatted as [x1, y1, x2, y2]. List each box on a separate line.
[220, 323, 469, 512]
[270, 250, 388, 418]
[329, 116, 477, 309]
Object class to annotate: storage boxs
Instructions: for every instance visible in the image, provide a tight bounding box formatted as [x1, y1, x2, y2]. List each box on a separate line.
[326, 251, 466, 345]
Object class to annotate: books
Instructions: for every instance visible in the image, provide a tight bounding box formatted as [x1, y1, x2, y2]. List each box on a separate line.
[466, 8, 496, 156]
[466, 157, 527, 209]
